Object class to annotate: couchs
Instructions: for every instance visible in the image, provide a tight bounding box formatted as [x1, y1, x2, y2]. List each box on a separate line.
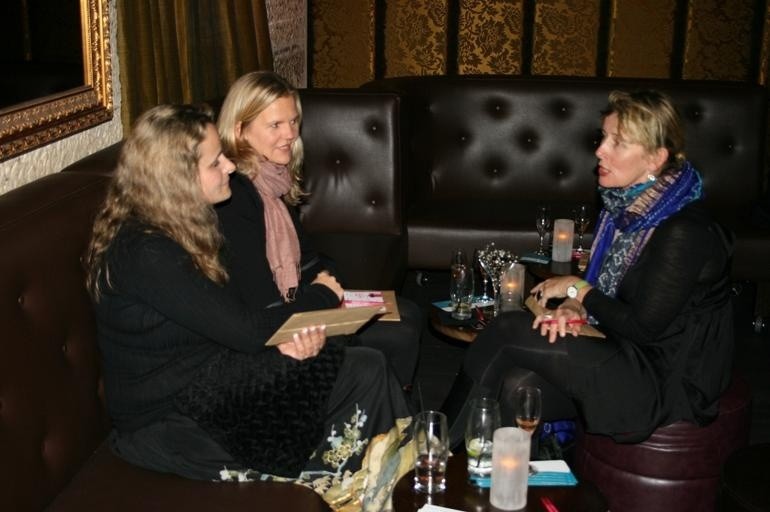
[359, 75, 769, 317]
[0, 89, 408, 512]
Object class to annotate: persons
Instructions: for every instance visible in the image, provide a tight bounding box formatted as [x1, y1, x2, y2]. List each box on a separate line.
[435, 86, 735, 453]
[81, 102, 413, 488]
[212, 69, 424, 393]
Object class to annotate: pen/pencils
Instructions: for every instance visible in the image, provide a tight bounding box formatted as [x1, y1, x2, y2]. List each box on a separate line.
[369, 294, 385, 297]
[342, 300, 388, 305]
[539, 319, 590, 324]
[475, 307, 486, 320]
[540, 495, 561, 512]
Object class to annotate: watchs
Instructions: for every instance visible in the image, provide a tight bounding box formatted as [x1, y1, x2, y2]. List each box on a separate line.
[566, 279, 591, 300]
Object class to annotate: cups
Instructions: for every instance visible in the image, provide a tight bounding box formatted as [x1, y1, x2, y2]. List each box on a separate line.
[412, 411, 446, 494]
[451, 265, 474, 320]
[463, 399, 502, 480]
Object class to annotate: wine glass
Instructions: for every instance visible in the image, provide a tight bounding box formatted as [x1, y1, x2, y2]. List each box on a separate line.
[473, 239, 496, 309]
[572, 205, 589, 254]
[516, 386, 544, 477]
[535, 207, 550, 256]
[479, 250, 516, 320]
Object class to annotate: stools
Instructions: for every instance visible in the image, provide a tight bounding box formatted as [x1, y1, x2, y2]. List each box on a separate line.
[715, 443, 770, 512]
[573, 367, 752, 512]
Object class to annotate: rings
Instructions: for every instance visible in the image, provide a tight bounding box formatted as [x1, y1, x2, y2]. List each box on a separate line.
[544, 312, 553, 319]
